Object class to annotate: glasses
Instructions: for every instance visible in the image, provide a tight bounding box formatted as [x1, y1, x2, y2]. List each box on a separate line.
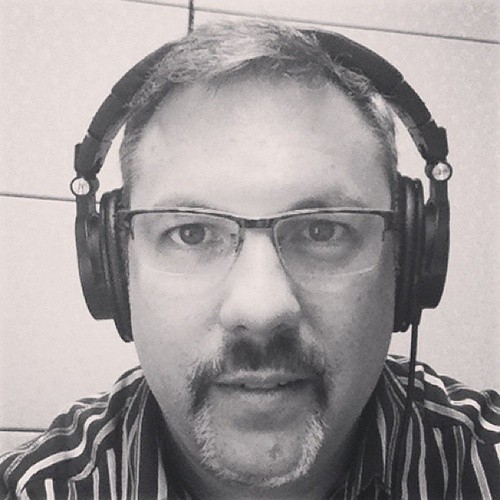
[118, 204, 402, 280]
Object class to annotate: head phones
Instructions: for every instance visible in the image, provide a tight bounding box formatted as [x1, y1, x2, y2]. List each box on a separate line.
[69, 30, 456, 343]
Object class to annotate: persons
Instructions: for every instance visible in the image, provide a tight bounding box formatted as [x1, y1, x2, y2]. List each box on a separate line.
[0, 13, 500, 500]
